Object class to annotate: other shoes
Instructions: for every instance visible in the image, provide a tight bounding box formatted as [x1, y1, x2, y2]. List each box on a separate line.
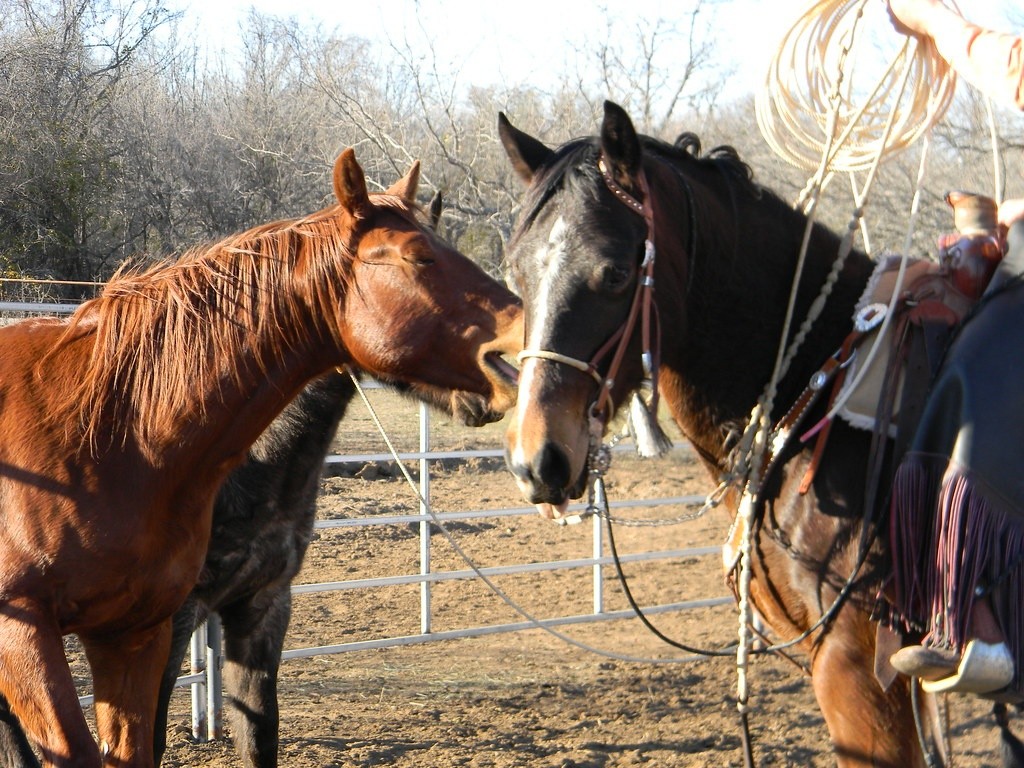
[890, 645, 960, 681]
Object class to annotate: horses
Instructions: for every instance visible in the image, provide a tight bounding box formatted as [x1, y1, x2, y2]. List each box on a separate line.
[3, 148, 524, 767]
[498, 103, 1022, 768]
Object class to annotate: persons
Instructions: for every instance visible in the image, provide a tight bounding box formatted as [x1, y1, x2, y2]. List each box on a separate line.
[885, 0, 1024, 682]
